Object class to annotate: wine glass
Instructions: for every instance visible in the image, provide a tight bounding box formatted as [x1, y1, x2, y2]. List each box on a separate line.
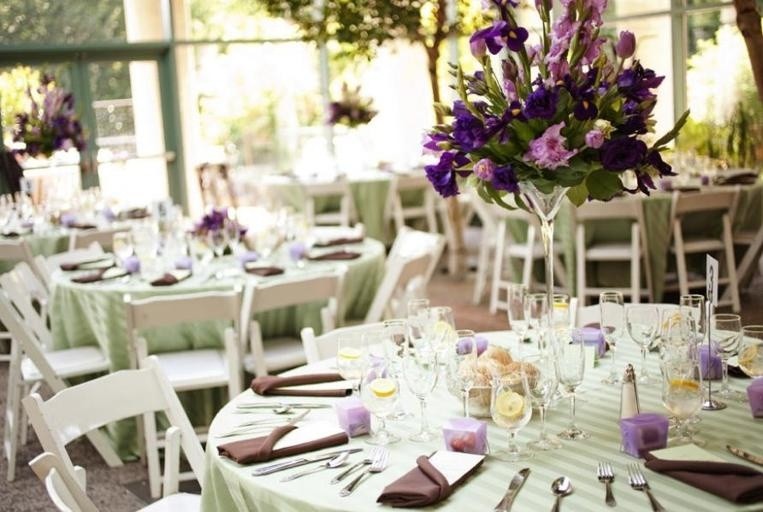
[678, 293, 707, 359]
[599, 290, 626, 386]
[659, 361, 708, 448]
[417, 306, 458, 369]
[506, 283, 532, 356]
[520, 350, 564, 452]
[710, 313, 745, 398]
[406, 297, 430, 350]
[737, 324, 763, 380]
[659, 319, 698, 375]
[361, 330, 392, 381]
[446, 329, 478, 418]
[0, 185, 103, 236]
[489, 369, 534, 463]
[401, 347, 439, 444]
[335, 333, 368, 399]
[522, 292, 552, 356]
[112, 204, 324, 289]
[552, 328, 592, 441]
[362, 376, 402, 446]
[634, 144, 722, 190]
[626, 305, 659, 385]
[382, 319, 410, 366]
[549, 294, 571, 329]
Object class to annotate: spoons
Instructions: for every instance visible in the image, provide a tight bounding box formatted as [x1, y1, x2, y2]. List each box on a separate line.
[234, 407, 291, 415]
[548, 475, 574, 512]
[279, 451, 349, 482]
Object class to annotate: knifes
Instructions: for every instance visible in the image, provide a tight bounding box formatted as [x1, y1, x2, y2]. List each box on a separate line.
[493, 468, 531, 512]
[726, 445, 763, 465]
[251, 448, 364, 477]
[236, 401, 331, 410]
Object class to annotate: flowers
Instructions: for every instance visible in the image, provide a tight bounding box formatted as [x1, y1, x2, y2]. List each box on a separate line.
[419, 0, 692, 219]
[2, 65, 89, 155]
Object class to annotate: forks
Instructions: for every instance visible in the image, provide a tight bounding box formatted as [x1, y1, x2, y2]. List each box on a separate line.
[340, 451, 391, 497]
[329, 449, 389, 498]
[597, 461, 618, 506]
[212, 408, 312, 438]
[234, 409, 305, 429]
[626, 462, 666, 511]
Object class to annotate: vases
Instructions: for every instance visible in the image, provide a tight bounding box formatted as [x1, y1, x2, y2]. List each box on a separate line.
[511, 176, 570, 406]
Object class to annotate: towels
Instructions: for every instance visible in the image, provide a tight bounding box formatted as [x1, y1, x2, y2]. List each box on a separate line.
[129, 209, 149, 219]
[150, 269, 192, 286]
[644, 445, 763, 506]
[1, 227, 33, 237]
[68, 222, 96, 230]
[70, 270, 104, 283]
[376, 451, 486, 509]
[250, 373, 353, 395]
[217, 425, 349, 465]
[245, 264, 283, 277]
[312, 238, 364, 247]
[583, 323, 614, 334]
[660, 181, 699, 193]
[308, 251, 360, 260]
[60, 259, 106, 270]
[713, 173, 759, 186]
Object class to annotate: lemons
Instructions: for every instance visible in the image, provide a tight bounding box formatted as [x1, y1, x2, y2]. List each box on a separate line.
[371, 378, 397, 397]
[740, 344, 756, 365]
[497, 392, 525, 418]
[673, 379, 700, 393]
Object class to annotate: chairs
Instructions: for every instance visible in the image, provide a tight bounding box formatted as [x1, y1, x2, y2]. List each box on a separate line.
[0, 269, 123, 479]
[296, 326, 383, 362]
[29, 448, 80, 510]
[674, 181, 742, 317]
[383, 227, 450, 284]
[245, 271, 347, 380]
[343, 174, 394, 235]
[13, 262, 52, 338]
[393, 175, 438, 240]
[119, 283, 245, 496]
[576, 190, 646, 306]
[372, 252, 434, 324]
[19, 354, 210, 511]
